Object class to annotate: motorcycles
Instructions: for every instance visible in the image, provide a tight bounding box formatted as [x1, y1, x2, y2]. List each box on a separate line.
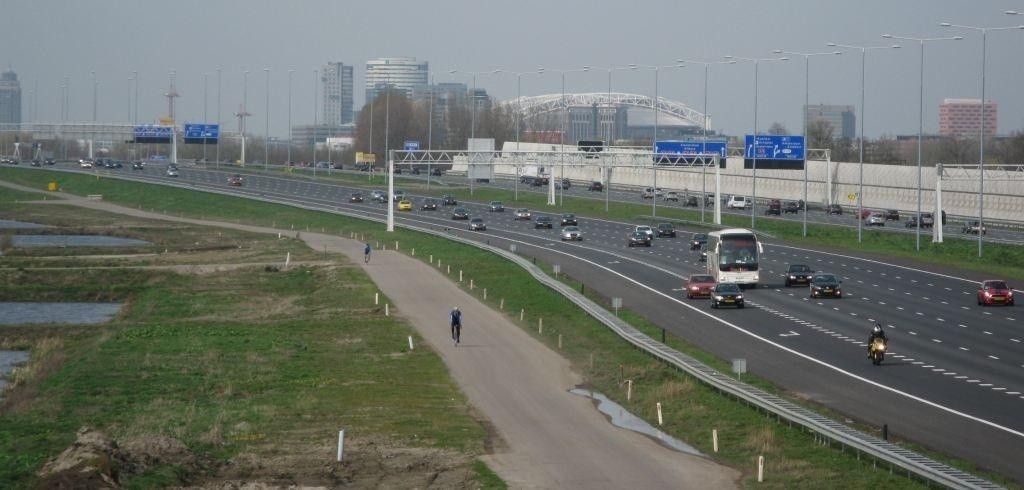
[866, 337, 889, 365]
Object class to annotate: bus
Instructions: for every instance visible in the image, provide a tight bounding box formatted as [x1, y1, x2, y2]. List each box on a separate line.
[706, 228, 764, 290]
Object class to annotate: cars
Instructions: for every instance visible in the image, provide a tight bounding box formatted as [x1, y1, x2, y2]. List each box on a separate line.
[629, 222, 707, 265]
[166, 163, 179, 178]
[349, 176, 603, 242]
[808, 272, 842, 299]
[709, 281, 744, 310]
[228, 173, 243, 186]
[688, 273, 718, 301]
[386, 161, 441, 176]
[644, 184, 808, 217]
[785, 263, 815, 286]
[77, 156, 146, 172]
[1, 155, 56, 168]
[977, 278, 1015, 308]
[828, 203, 987, 238]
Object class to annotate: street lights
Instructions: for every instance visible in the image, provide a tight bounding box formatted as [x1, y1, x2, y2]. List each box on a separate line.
[1005, 11, 1024, 16]
[677, 56, 732, 225]
[880, 34, 963, 254]
[199, 64, 391, 176]
[629, 63, 680, 217]
[726, 55, 781, 233]
[24, 67, 180, 159]
[772, 48, 836, 236]
[826, 42, 902, 241]
[423, 63, 641, 213]
[939, 22, 1023, 261]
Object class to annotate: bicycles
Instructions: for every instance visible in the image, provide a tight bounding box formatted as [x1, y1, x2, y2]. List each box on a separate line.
[451, 325, 459, 348]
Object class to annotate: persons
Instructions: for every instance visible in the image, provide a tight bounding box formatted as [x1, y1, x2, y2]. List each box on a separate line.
[364, 243, 371, 264]
[448, 306, 462, 345]
[866, 325, 886, 358]
